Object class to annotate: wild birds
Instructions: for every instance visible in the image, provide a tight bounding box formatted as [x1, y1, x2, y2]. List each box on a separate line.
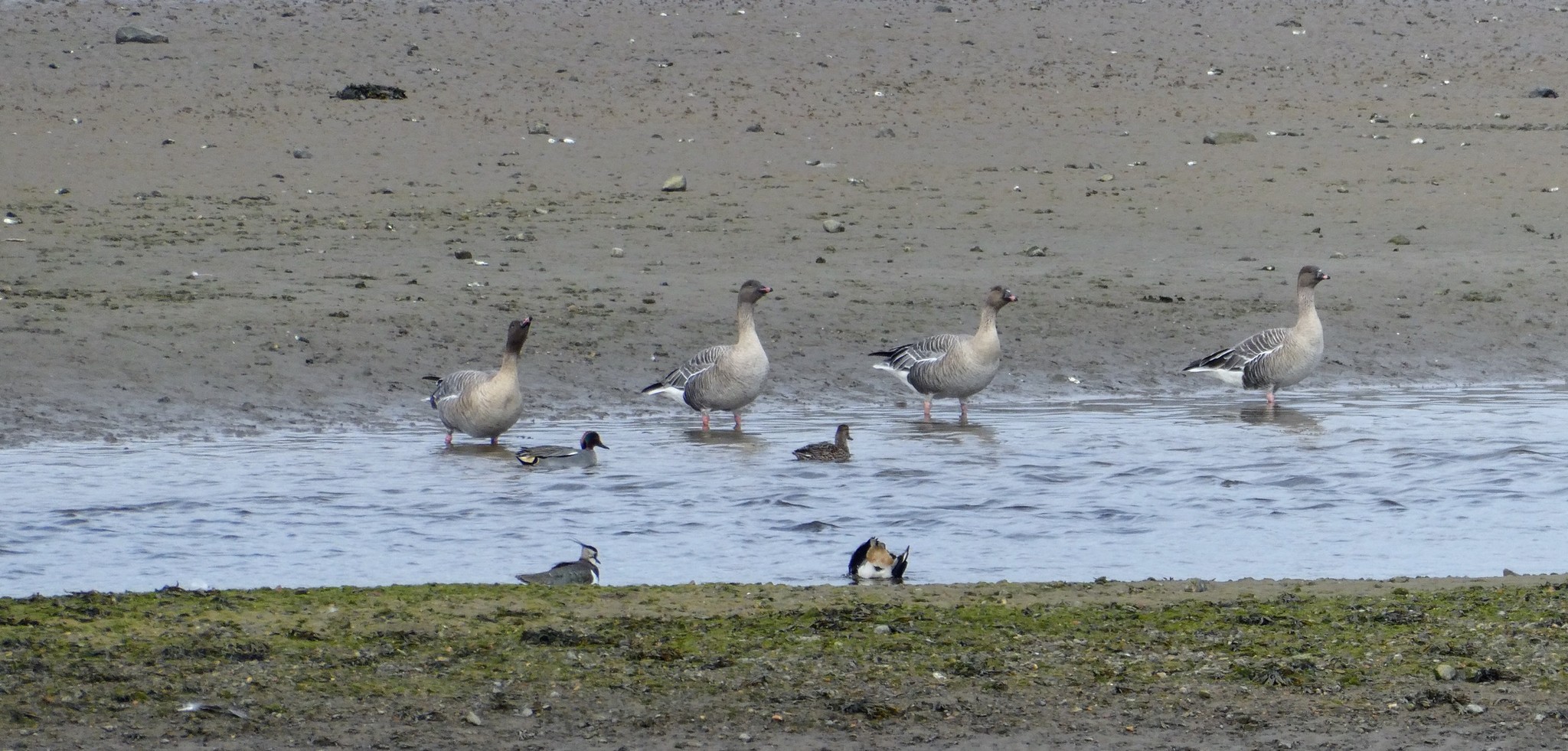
[642, 279, 773, 432]
[516, 537, 602, 585]
[868, 286, 1018, 422]
[792, 424, 853, 464]
[420, 316, 535, 443]
[1184, 265, 1332, 404]
[849, 536, 910, 579]
[515, 431, 612, 470]
